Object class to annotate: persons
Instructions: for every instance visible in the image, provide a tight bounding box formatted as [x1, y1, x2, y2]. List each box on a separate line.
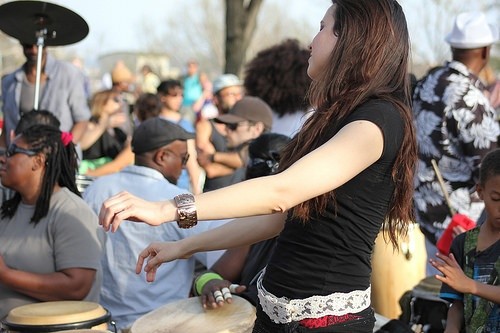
[0, 40, 277, 333]
[429, 149, 500, 333]
[99, 0, 416, 333]
[411, 13, 500, 243]
[369, 215, 447, 333]
[193, 239, 277, 310]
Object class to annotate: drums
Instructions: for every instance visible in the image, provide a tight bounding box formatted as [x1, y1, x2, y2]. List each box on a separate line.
[0, 300, 111, 333]
[370, 218, 428, 321]
[126, 294, 257, 333]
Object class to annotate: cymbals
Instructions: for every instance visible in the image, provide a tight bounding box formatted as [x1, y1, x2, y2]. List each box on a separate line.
[0, 0, 89, 46]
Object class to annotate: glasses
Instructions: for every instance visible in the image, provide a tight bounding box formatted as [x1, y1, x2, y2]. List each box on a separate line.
[5, 142, 37, 157]
[225, 122, 252, 130]
[112, 96, 128, 103]
[167, 149, 190, 165]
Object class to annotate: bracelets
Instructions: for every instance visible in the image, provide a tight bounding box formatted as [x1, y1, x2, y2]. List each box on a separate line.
[194, 272, 223, 296]
[174, 194, 197, 228]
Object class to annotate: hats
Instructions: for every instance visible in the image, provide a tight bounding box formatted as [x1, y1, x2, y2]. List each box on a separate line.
[130, 118, 195, 153]
[210, 96, 273, 132]
[213, 74, 245, 94]
[444, 10, 499, 49]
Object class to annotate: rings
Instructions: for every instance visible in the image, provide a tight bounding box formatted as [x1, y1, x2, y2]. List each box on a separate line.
[222, 287, 230, 295]
[216, 296, 223, 303]
[214, 291, 222, 298]
[224, 293, 232, 300]
[229, 283, 239, 293]
